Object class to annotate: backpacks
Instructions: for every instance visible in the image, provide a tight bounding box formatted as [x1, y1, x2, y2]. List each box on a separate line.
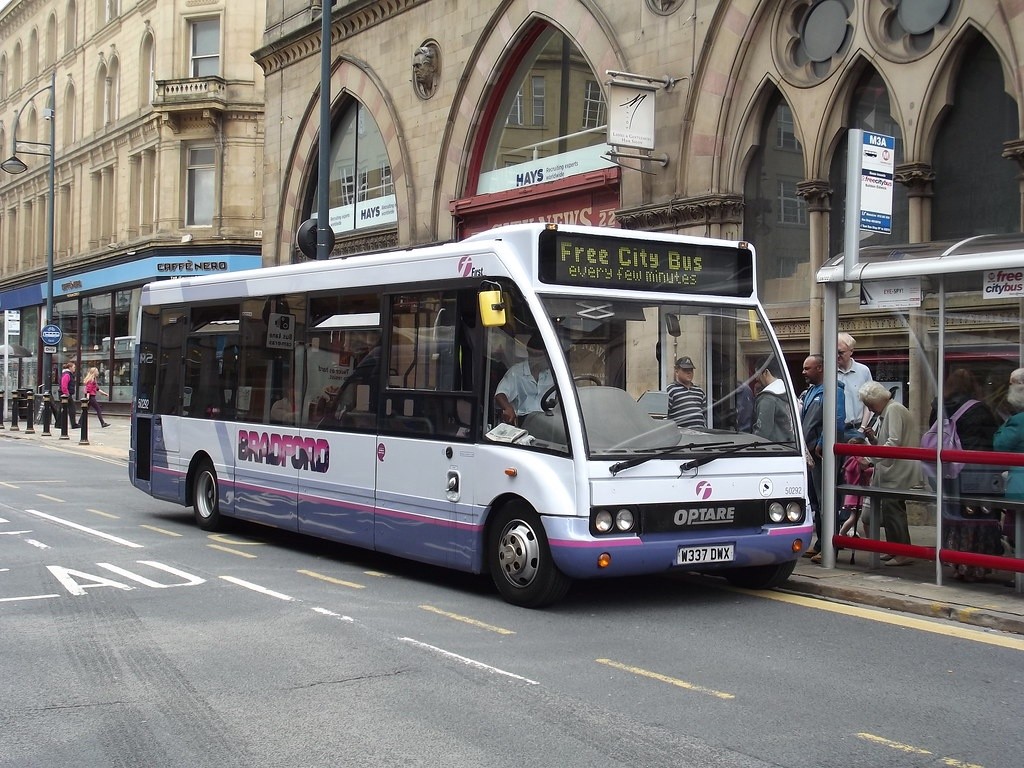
[920, 399, 981, 479]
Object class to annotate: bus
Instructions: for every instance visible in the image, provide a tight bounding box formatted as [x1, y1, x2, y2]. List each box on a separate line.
[128, 218, 817, 611]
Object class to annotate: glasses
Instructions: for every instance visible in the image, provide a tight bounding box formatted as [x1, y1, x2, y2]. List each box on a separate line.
[838, 349, 849, 355]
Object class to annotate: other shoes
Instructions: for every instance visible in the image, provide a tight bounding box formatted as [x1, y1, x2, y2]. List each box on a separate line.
[880, 554, 916, 566]
[1005, 579, 1015, 588]
[72, 424, 81, 429]
[947, 574, 983, 583]
[811, 552, 838, 564]
[801, 549, 819, 558]
[102, 423, 111, 427]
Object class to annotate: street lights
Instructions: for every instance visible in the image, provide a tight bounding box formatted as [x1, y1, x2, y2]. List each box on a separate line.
[2, 75, 55, 424]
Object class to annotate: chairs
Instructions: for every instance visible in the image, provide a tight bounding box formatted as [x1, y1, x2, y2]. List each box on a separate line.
[262, 358, 290, 424]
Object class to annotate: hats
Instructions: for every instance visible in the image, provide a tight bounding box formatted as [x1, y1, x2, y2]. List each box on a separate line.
[674, 356, 696, 369]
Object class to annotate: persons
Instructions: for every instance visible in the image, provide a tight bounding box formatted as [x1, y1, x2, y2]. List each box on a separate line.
[736, 380, 754, 434]
[837, 332, 872, 433]
[269, 375, 300, 422]
[335, 336, 384, 420]
[838, 437, 874, 537]
[993, 367, 1024, 554]
[78, 367, 111, 428]
[921, 368, 1006, 584]
[753, 354, 794, 445]
[666, 355, 708, 430]
[802, 354, 847, 565]
[55, 363, 83, 429]
[495, 334, 557, 428]
[859, 380, 920, 566]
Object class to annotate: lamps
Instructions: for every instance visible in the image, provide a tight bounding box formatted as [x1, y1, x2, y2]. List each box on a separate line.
[181, 234, 192, 243]
